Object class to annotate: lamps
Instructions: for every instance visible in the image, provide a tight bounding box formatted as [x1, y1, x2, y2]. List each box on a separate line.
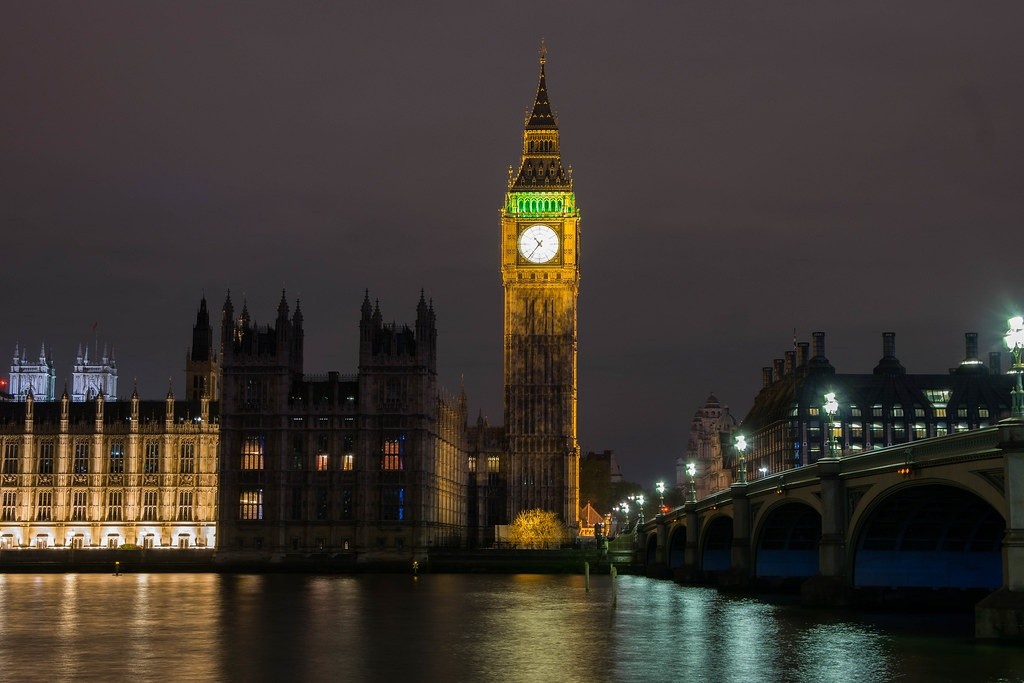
[777, 476, 784, 494]
[898, 447, 912, 474]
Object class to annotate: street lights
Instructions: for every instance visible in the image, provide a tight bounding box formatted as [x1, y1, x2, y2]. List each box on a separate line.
[823, 393, 838, 457]
[759, 468, 767, 477]
[655, 482, 665, 514]
[612, 495, 645, 532]
[684, 463, 697, 501]
[733, 435, 747, 483]
[1002, 316, 1024, 418]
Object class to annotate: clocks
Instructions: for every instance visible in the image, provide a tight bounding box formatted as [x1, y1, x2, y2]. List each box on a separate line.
[516, 223, 560, 264]
